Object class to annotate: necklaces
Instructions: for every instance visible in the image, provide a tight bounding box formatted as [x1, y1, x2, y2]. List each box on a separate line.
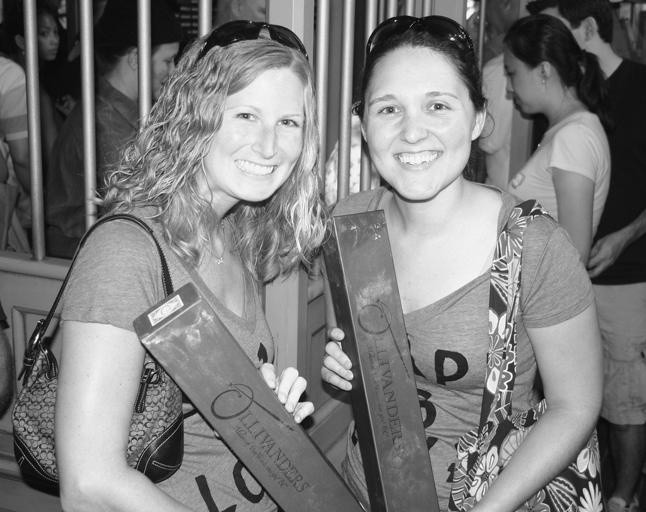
[204, 216, 226, 265]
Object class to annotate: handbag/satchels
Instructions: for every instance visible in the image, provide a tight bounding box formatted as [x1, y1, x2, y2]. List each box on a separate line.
[10, 212, 185, 498]
[451, 395, 606, 512]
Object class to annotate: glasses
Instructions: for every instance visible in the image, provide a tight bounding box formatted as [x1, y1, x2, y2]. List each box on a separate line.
[194, 19, 311, 63]
[365, 15, 477, 67]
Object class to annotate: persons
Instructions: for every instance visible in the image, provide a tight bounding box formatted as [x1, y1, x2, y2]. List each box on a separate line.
[1, 304, 14, 416]
[321, 14, 606, 512]
[470, 0, 645, 512]
[1, 0, 187, 259]
[55, 21, 333, 512]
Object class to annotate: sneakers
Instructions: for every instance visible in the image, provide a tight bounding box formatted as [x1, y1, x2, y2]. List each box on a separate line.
[607, 493, 641, 512]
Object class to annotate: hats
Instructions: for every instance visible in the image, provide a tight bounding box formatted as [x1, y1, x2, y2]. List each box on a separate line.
[93, 0, 190, 44]
